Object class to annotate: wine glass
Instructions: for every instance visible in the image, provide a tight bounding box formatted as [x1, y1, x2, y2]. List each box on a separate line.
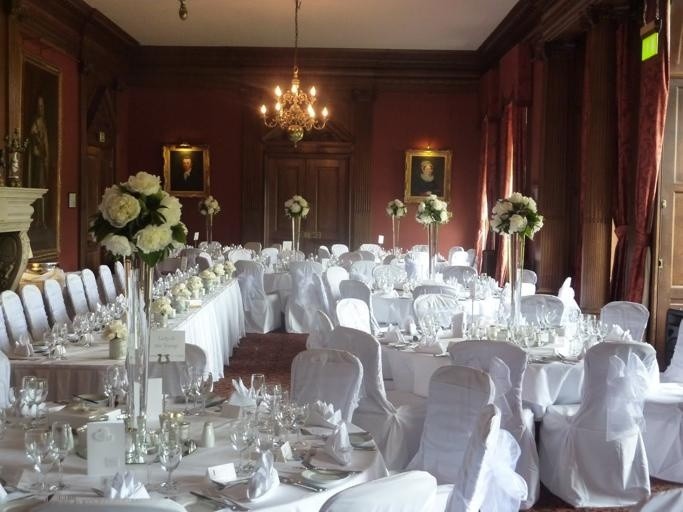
[418, 306, 603, 347]
[6, 364, 308, 492]
[41, 262, 208, 361]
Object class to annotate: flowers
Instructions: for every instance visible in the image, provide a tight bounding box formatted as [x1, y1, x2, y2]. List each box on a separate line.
[198, 196, 221, 216]
[415, 194, 453, 224]
[490, 191, 545, 242]
[384, 198, 408, 218]
[90, 170, 188, 267]
[284, 194, 310, 219]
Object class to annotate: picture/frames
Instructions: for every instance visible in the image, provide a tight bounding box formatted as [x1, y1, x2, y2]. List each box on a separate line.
[163, 144, 211, 198]
[7, 44, 65, 263]
[403, 148, 453, 205]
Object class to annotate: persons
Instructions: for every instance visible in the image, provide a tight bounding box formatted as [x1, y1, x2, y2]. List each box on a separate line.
[25, 93, 50, 231]
[412, 160, 442, 196]
[171, 156, 202, 191]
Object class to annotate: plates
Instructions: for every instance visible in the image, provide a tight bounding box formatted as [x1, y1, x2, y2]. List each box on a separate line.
[390, 341, 416, 350]
[302, 469, 349, 483]
[172, 494, 226, 511]
[1, 499, 45, 512]
[324, 432, 375, 446]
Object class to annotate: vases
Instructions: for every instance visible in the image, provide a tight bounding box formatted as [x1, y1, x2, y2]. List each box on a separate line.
[206, 215, 213, 245]
[392, 213, 400, 255]
[291, 214, 302, 251]
[428, 221, 438, 280]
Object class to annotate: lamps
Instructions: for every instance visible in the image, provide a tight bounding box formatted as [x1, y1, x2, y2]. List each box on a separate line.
[425, 144, 431, 152]
[260, 0, 331, 147]
[179, 139, 191, 146]
